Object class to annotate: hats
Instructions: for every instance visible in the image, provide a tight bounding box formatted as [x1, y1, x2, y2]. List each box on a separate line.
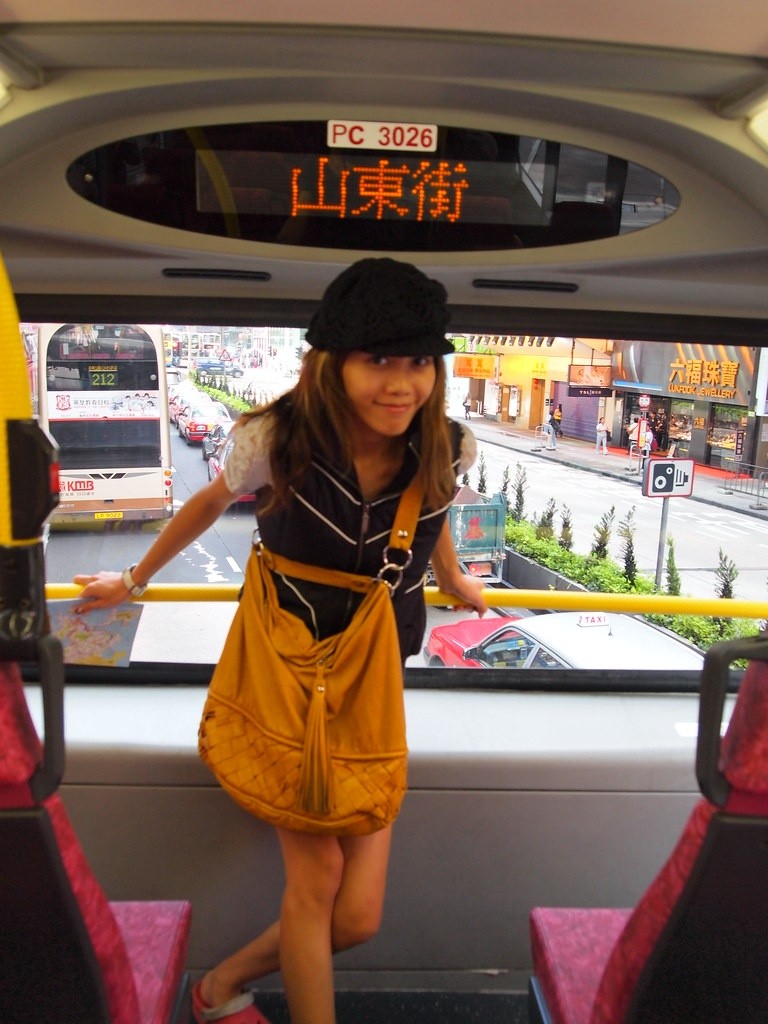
[305, 257, 455, 357]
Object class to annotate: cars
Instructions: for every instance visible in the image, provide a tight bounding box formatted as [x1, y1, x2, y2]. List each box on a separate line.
[202, 422, 236, 461]
[168, 392, 213, 429]
[423, 611, 705, 670]
[194, 360, 244, 378]
[205, 440, 257, 502]
[177, 402, 232, 446]
[165, 368, 198, 406]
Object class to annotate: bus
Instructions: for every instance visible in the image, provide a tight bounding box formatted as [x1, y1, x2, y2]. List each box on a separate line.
[38, 323, 173, 527]
[161, 333, 173, 367]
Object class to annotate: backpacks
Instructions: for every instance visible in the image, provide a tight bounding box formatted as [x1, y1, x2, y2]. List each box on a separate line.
[648, 431, 659, 450]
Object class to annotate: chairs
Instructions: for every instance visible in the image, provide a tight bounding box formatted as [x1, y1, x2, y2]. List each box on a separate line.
[58, 343, 145, 359]
[529, 633, 768, 1024]
[0, 636, 194, 1024]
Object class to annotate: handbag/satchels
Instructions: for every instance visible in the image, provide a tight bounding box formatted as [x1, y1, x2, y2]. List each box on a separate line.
[627, 427, 633, 434]
[198, 543, 409, 836]
[606, 430, 613, 441]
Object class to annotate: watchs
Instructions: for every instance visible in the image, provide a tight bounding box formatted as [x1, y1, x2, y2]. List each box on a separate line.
[121, 564, 147, 596]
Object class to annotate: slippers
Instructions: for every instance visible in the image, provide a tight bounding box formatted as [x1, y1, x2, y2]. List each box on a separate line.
[191, 980, 270, 1024]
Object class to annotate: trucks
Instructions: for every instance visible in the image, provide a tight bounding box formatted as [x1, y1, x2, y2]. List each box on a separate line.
[425, 484, 507, 610]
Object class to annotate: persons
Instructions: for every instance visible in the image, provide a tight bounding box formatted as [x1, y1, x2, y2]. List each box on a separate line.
[124, 393, 155, 412]
[641, 424, 653, 473]
[547, 404, 563, 436]
[74, 258, 484, 1024]
[595, 417, 609, 455]
[175, 356, 179, 368]
[625, 411, 667, 456]
[463, 392, 471, 420]
